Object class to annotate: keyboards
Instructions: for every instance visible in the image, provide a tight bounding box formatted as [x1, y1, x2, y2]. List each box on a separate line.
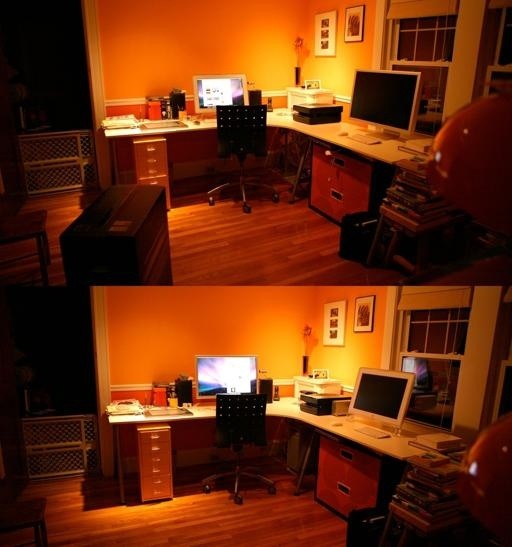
[355, 427, 390, 439]
[346, 133, 381, 145]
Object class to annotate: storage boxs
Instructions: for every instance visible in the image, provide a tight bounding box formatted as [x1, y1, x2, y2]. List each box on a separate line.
[297, 390, 352, 415]
[285, 79, 333, 110]
[332, 399, 351, 416]
[294, 368, 343, 402]
[292, 104, 344, 125]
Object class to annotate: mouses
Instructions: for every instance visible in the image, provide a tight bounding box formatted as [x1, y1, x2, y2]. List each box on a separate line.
[337, 131, 348, 136]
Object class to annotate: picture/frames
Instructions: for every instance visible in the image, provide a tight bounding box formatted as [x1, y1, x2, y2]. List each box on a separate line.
[343, 4, 364, 42]
[353, 294, 376, 333]
[322, 298, 346, 347]
[313, 9, 337, 58]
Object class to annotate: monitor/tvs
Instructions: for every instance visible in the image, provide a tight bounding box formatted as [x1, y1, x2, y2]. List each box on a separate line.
[348, 367, 416, 429]
[194, 355, 258, 400]
[192, 73, 249, 123]
[348, 68, 424, 139]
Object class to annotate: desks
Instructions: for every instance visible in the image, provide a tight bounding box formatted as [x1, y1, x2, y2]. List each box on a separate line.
[104, 396, 467, 522]
[100, 105, 438, 238]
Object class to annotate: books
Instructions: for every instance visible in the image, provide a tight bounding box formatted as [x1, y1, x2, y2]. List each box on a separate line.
[101, 113, 138, 130]
[105, 399, 145, 416]
[388, 432, 473, 534]
[378, 137, 465, 233]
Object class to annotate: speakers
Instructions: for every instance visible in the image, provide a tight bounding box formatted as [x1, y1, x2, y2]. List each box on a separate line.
[169, 92, 186, 119]
[259, 379, 273, 403]
[248, 90, 262, 106]
[175, 380, 192, 406]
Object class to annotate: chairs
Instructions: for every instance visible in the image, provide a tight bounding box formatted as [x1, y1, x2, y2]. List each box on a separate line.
[206, 103, 280, 212]
[201, 392, 279, 502]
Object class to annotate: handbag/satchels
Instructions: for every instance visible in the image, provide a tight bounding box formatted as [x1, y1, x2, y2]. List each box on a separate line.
[339, 211, 380, 263]
[347, 508, 389, 546]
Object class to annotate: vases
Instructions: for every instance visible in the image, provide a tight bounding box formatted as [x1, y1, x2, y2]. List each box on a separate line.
[302, 356, 308, 375]
[295, 67, 301, 86]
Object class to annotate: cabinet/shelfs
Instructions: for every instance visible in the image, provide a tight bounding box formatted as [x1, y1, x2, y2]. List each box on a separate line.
[309, 141, 372, 226]
[114, 136, 173, 212]
[112, 421, 174, 503]
[314, 427, 404, 522]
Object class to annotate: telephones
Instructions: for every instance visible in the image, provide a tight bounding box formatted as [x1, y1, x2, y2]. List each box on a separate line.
[266, 97, 273, 112]
[273, 386, 280, 401]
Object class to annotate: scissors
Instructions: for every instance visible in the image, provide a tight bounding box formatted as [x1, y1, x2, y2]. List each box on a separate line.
[166, 390, 177, 398]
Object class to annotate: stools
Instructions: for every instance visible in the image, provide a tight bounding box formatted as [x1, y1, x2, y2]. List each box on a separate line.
[1, 209, 53, 290]
[1, 496, 52, 547]
